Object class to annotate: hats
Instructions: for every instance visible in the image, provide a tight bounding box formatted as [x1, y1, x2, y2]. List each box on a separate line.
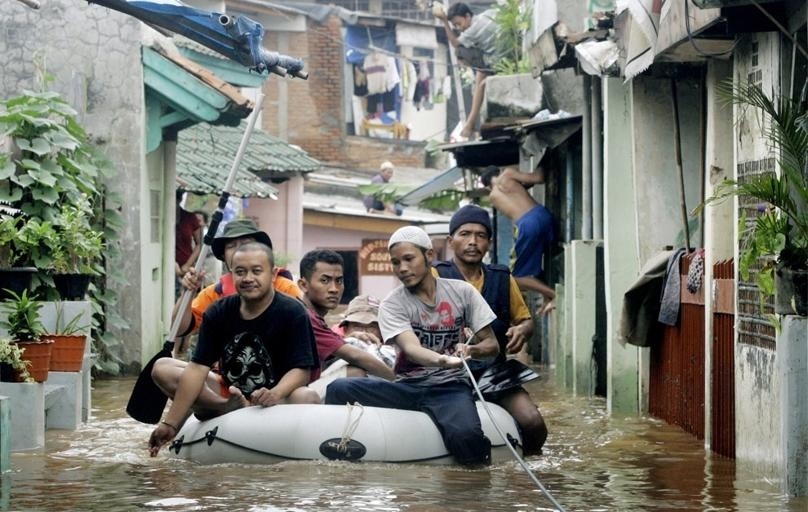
[337, 293, 383, 328]
[449, 204, 491, 234]
[388, 226, 433, 251]
[213, 219, 271, 259]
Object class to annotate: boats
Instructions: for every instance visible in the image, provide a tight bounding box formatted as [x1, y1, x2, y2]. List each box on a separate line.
[161, 400, 524, 466]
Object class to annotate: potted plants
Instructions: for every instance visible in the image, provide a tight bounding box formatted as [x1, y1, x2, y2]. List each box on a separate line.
[48, 192, 104, 300]
[0, 285, 57, 382]
[687, 70, 808, 317]
[0, 210, 59, 299]
[40, 298, 90, 375]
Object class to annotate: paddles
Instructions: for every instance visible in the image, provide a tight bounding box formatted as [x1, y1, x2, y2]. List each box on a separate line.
[471, 358, 541, 401]
[127, 93, 266, 424]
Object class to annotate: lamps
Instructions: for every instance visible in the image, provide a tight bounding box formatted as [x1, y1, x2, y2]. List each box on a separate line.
[572, 38, 621, 77]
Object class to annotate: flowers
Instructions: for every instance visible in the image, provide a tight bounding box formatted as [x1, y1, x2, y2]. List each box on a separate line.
[0, 337, 35, 383]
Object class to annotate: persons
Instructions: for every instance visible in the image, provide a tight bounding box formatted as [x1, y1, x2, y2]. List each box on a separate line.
[481, 163, 561, 318]
[432, 1, 521, 140]
[361, 161, 403, 217]
[149, 187, 550, 469]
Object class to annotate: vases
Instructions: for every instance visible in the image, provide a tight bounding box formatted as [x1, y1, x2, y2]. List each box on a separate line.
[1, 362, 17, 382]
